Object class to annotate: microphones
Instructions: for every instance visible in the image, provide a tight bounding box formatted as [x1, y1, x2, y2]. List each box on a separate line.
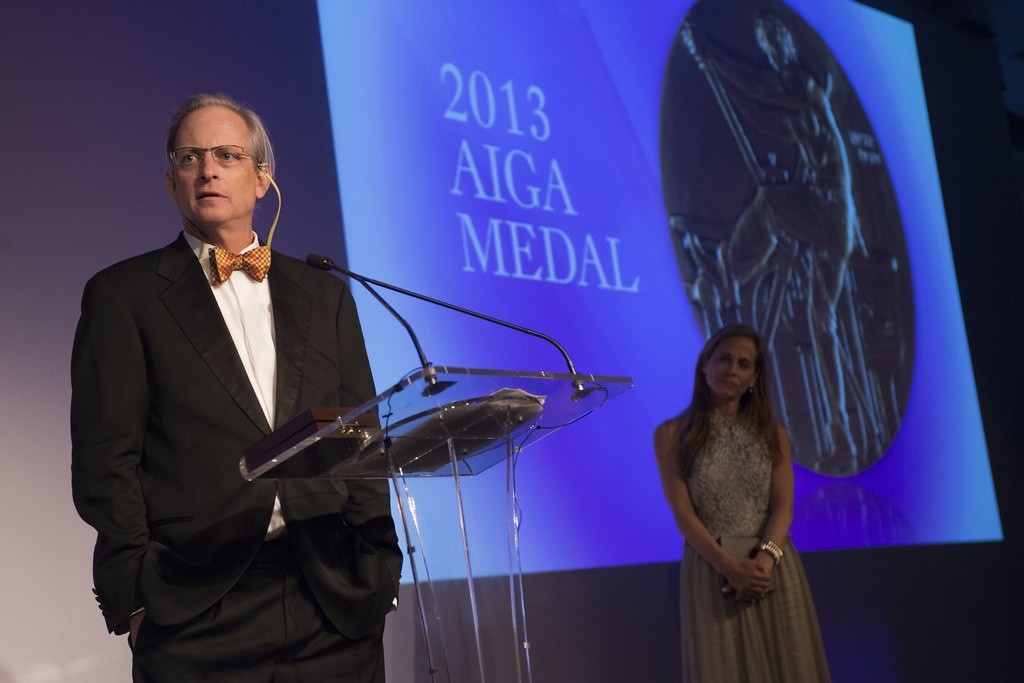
[305, 254, 586, 390]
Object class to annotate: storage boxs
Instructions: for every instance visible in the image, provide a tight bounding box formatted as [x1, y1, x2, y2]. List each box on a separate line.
[243, 405, 384, 480]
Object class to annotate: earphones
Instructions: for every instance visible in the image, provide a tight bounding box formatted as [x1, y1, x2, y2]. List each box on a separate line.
[169, 177, 176, 195]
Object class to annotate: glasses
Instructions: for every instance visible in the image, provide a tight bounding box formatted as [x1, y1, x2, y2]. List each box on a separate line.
[169, 145, 262, 165]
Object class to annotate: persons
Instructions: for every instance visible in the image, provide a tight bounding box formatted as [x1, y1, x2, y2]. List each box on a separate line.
[655, 324, 830, 681]
[669, 12, 855, 336]
[69, 92, 402, 682]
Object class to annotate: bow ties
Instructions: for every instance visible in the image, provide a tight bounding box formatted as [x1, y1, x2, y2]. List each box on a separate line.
[209, 245, 272, 285]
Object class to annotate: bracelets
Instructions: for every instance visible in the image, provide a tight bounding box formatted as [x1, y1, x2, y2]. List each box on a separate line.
[758, 541, 783, 564]
